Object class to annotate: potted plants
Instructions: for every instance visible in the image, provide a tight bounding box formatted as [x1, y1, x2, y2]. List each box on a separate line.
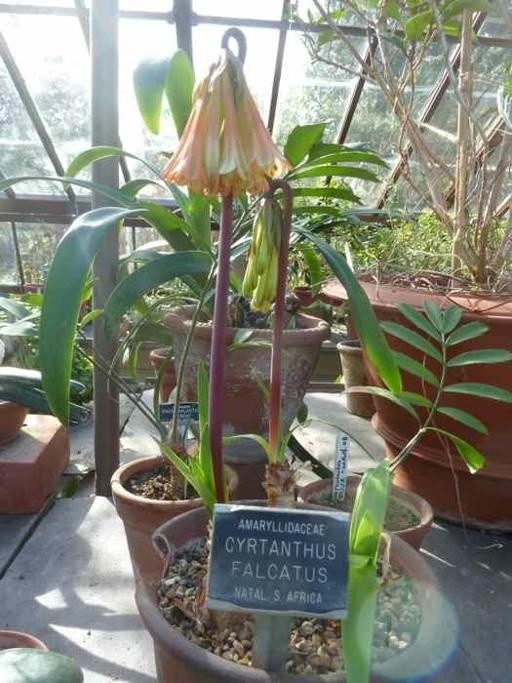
[0, 0, 512, 681]
